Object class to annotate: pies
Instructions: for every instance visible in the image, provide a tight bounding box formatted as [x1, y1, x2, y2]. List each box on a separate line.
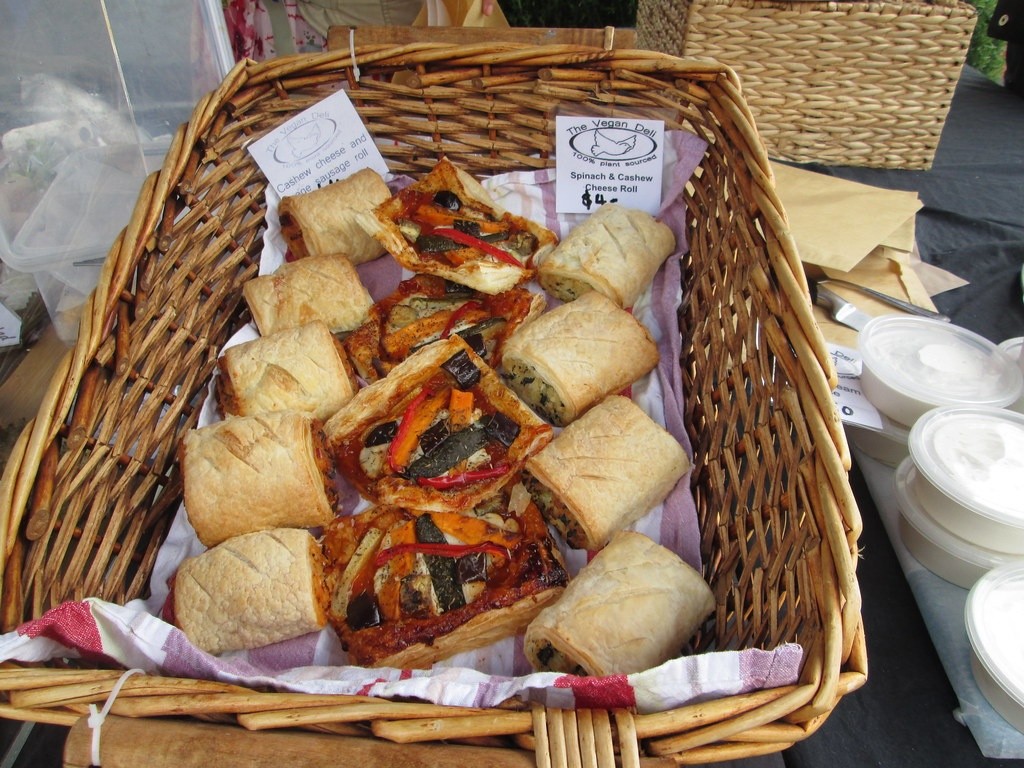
[499, 202, 716, 681]
[163, 166, 395, 655]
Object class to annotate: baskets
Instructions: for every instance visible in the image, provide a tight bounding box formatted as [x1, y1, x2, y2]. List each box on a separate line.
[0, 40, 870, 768]
[634, 0, 979, 172]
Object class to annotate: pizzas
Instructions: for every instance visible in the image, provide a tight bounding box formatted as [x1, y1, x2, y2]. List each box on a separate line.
[315, 157, 569, 673]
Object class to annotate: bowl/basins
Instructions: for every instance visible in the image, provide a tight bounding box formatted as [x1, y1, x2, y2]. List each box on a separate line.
[858, 314, 1024, 737]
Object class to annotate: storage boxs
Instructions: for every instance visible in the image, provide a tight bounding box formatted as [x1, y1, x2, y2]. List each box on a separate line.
[0, 0, 235, 351]
